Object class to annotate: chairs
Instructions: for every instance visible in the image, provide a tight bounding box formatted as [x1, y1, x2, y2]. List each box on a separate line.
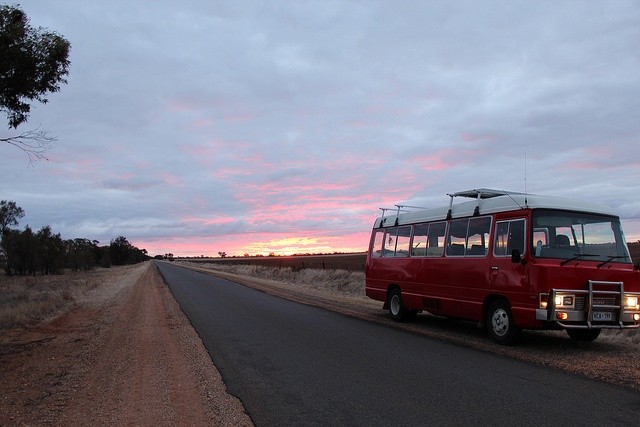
[450, 244, 463, 254]
[471, 245, 481, 255]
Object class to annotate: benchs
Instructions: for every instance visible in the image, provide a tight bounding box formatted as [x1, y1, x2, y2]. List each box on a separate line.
[412, 248, 425, 256]
[428, 247, 443, 256]
[540, 235, 580, 257]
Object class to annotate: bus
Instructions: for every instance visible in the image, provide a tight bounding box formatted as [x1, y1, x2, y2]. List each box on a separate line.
[365, 188, 639, 345]
